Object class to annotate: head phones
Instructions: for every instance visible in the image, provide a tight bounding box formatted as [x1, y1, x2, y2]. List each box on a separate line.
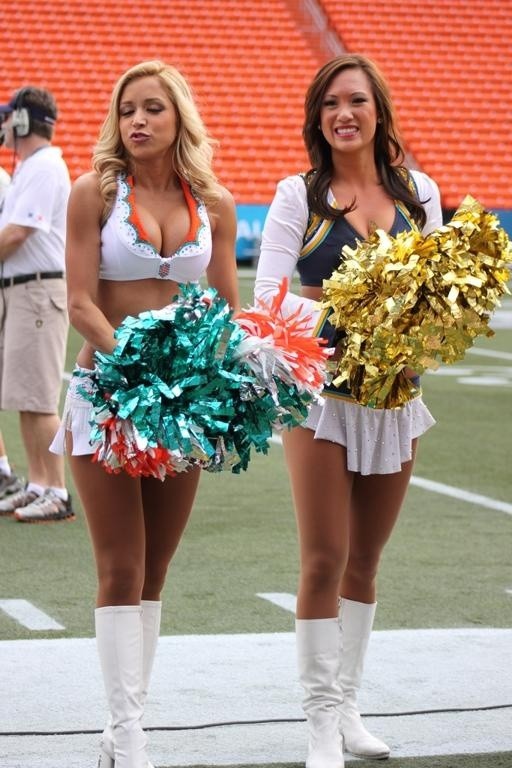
[12, 88, 31, 137]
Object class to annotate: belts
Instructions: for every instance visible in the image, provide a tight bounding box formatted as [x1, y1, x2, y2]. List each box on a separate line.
[0, 272, 62, 289]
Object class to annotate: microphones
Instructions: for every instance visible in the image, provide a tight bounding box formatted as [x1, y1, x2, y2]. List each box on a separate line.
[0, 120, 21, 139]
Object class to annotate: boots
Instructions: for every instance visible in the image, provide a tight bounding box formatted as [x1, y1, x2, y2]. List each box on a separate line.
[339, 595, 390, 760]
[294, 617, 344, 768]
[94, 600, 162, 768]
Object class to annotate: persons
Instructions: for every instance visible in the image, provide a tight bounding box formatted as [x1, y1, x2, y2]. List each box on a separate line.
[0, 85, 76, 524]
[0, 104, 21, 499]
[252, 54, 443, 765]
[48, 59, 243, 768]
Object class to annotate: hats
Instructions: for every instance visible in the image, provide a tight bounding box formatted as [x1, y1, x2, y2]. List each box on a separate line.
[0, 86, 56, 123]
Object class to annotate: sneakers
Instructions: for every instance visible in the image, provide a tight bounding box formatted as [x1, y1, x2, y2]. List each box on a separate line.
[0, 468, 75, 524]
